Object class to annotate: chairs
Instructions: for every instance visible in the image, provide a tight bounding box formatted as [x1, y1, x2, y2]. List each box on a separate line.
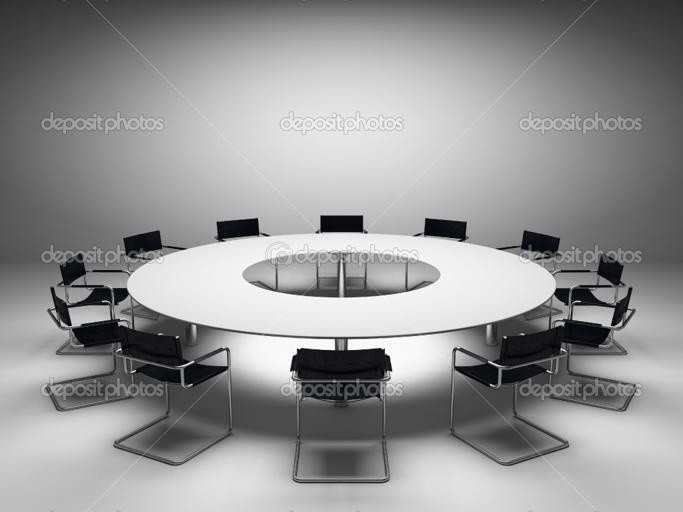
[44, 215, 637, 484]
